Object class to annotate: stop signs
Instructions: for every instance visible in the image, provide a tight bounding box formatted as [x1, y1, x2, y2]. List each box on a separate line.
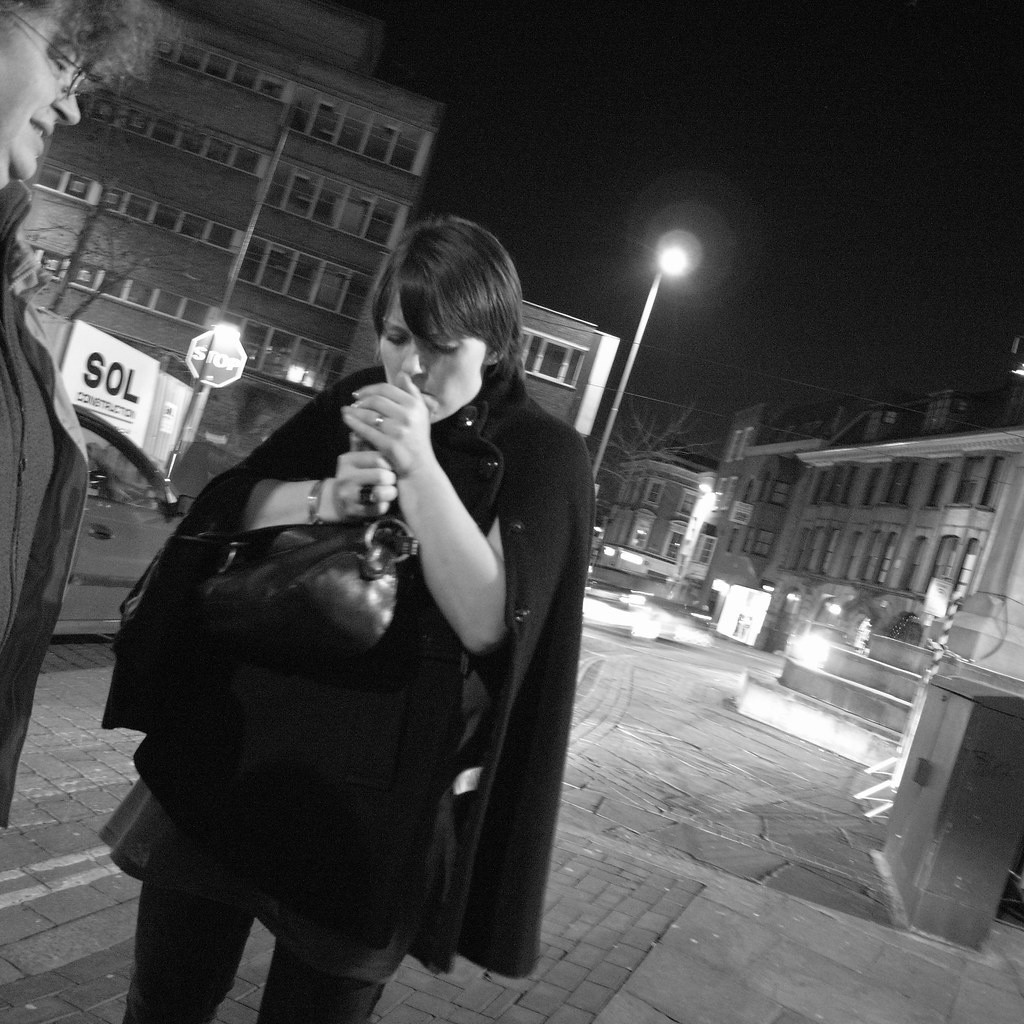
[184, 328, 248, 388]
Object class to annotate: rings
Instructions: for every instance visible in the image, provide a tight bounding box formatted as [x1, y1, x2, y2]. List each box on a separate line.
[358, 484, 375, 505]
[375, 416, 386, 429]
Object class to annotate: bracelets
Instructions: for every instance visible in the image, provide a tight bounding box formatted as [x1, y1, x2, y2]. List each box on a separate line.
[308, 478, 326, 525]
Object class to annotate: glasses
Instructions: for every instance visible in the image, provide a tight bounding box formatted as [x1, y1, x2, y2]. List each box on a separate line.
[0, 0, 90, 102]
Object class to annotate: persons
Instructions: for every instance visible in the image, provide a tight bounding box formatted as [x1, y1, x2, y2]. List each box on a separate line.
[95, 216, 592, 1024]
[0, 0, 168, 823]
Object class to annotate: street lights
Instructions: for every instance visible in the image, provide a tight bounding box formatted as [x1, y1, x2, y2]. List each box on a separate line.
[592, 246, 692, 480]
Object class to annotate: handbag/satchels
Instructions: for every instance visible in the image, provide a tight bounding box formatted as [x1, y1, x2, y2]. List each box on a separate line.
[194, 504, 415, 660]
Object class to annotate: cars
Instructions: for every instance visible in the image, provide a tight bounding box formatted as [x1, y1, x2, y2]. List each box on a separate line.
[49, 403, 196, 635]
